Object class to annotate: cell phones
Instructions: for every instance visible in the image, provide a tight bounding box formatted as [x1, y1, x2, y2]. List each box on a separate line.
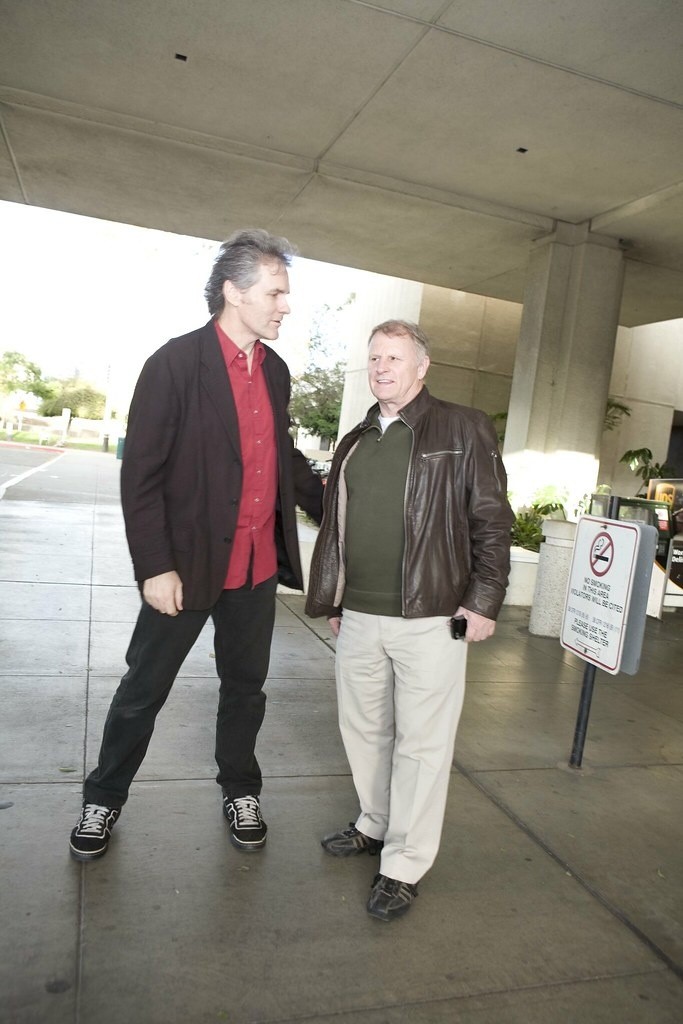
[451, 616, 468, 640]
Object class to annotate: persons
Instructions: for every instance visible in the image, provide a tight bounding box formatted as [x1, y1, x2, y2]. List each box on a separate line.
[67, 229, 327, 862]
[304, 320, 514, 922]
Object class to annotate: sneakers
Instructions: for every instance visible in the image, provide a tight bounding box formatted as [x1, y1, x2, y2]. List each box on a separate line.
[223, 793, 268, 851]
[366, 872, 420, 921]
[69, 799, 121, 861]
[321, 822, 384, 857]
